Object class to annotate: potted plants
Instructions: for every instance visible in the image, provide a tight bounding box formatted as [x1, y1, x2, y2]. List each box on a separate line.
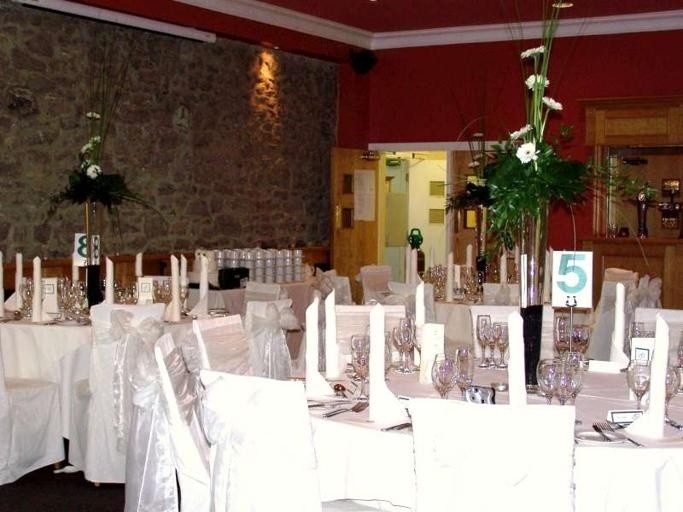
[630, 181, 658, 239]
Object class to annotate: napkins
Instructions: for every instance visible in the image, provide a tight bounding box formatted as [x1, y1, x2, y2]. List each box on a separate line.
[493, 251, 511, 304]
[368, 299, 413, 430]
[91, 254, 115, 304]
[177, 254, 189, 288]
[2, 251, 23, 313]
[31, 254, 53, 321]
[445, 250, 456, 302]
[466, 242, 475, 266]
[625, 312, 672, 438]
[164, 254, 181, 322]
[323, 284, 351, 379]
[136, 251, 159, 302]
[413, 279, 427, 345]
[305, 293, 337, 398]
[186, 253, 209, 316]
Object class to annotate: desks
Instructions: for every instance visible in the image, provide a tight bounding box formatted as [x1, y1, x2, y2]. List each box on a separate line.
[0, 305, 296, 486]
[293, 361, 683, 511]
[174, 276, 357, 361]
[433, 297, 541, 379]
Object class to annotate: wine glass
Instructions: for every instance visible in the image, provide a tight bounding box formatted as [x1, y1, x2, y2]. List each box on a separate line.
[18, 278, 46, 319]
[351, 324, 371, 401]
[178, 279, 189, 314]
[477, 314, 508, 369]
[392, 318, 417, 374]
[57, 275, 88, 322]
[536, 317, 591, 424]
[619, 322, 683, 425]
[431, 348, 472, 399]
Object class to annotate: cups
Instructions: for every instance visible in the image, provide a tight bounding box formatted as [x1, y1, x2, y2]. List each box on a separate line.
[153, 277, 173, 307]
[213, 247, 306, 283]
[113, 280, 139, 304]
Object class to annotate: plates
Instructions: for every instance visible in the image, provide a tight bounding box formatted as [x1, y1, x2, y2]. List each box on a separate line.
[574, 430, 627, 444]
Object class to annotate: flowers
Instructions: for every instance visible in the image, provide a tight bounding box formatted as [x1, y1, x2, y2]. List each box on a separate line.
[39, 14, 169, 243]
[440, 0, 632, 263]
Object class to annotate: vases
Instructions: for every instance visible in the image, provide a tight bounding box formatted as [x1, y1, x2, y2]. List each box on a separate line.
[520, 204, 550, 393]
[81, 199, 103, 312]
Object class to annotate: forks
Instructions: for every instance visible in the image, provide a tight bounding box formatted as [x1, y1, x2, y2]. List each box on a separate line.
[593, 421, 642, 446]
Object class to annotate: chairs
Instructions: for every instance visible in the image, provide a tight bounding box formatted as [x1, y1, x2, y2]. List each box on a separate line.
[467, 304, 519, 358]
[68, 300, 166, 487]
[243, 280, 282, 302]
[557, 266, 663, 367]
[138, 274, 190, 303]
[385, 280, 437, 367]
[190, 313, 267, 378]
[359, 264, 392, 307]
[0, 354, 66, 485]
[154, 332, 213, 512]
[195, 365, 385, 512]
[244, 296, 296, 378]
[408, 395, 577, 512]
[634, 307, 682, 368]
